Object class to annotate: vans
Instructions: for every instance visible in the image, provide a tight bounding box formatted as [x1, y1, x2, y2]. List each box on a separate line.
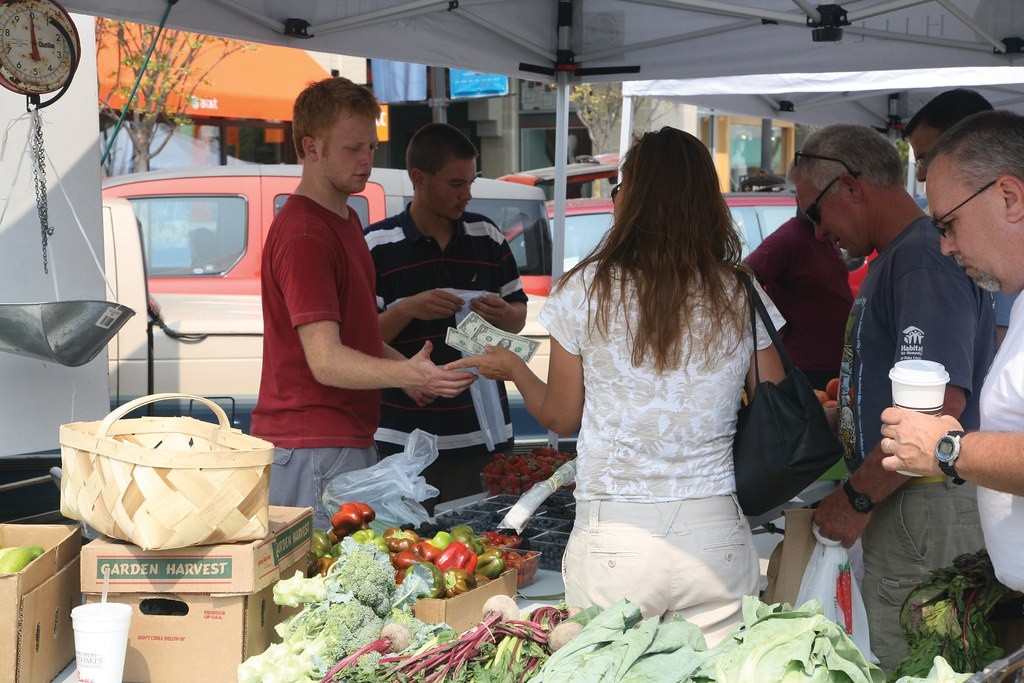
[543, 191, 799, 273]
[101, 166, 552, 436]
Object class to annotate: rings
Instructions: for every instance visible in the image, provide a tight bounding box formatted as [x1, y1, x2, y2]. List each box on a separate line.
[885, 439, 891, 451]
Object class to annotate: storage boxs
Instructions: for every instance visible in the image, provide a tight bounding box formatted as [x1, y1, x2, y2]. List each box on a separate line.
[0, 455, 574, 683]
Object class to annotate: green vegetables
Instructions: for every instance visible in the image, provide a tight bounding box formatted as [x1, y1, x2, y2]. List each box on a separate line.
[520, 597, 887, 683]
[886, 547, 1015, 683]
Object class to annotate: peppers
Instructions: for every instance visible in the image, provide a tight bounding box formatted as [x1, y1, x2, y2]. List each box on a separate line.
[305, 502, 505, 599]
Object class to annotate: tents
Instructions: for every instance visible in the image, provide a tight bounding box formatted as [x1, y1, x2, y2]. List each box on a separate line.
[616, 67, 1024, 189]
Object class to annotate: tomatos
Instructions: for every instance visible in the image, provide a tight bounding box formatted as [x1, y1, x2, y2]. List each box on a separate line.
[479, 530, 537, 589]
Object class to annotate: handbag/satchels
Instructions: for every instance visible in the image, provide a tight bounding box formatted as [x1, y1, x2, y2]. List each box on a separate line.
[733, 266, 844, 516]
[794, 522, 882, 665]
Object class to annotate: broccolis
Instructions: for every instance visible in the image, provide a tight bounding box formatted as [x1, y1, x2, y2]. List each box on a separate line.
[230, 543, 427, 683]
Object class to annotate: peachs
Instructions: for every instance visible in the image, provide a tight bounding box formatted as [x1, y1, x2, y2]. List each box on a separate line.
[813, 377, 855, 410]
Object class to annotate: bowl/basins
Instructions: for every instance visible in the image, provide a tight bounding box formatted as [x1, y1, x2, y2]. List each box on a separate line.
[434, 473, 576, 588]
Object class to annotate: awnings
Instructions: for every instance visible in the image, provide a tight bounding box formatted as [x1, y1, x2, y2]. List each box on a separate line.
[94, 16, 331, 121]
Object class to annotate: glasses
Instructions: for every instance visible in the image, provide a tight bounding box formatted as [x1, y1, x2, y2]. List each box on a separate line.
[930, 180, 997, 239]
[794, 150, 858, 179]
[804, 170, 863, 227]
[913, 159, 927, 171]
[610, 183, 623, 203]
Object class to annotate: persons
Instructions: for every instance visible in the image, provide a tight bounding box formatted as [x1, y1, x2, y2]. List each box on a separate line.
[248, 77, 479, 532]
[741, 88, 1024, 676]
[445, 125, 789, 647]
[362, 122, 529, 514]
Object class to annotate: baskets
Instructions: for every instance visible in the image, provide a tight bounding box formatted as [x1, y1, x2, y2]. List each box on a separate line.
[57, 392, 273, 549]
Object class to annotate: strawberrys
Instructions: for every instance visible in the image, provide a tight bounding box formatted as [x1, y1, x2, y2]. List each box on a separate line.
[483, 446, 577, 498]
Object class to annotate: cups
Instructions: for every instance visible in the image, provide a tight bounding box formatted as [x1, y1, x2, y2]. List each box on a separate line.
[69, 602, 133, 683]
[888, 358, 950, 477]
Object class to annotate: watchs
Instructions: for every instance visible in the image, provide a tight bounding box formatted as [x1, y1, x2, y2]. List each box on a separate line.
[934, 430, 969, 486]
[843, 480, 880, 513]
